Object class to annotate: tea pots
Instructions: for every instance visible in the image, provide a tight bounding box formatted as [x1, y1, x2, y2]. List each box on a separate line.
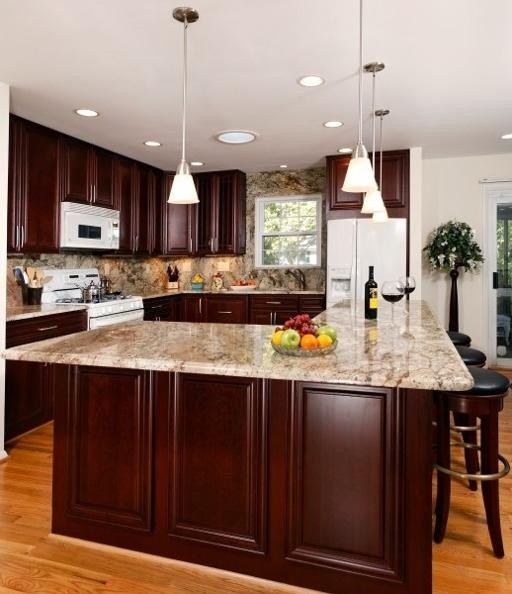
[74, 280, 105, 301]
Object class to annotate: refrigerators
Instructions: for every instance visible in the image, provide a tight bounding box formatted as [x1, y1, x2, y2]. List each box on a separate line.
[327, 218, 407, 309]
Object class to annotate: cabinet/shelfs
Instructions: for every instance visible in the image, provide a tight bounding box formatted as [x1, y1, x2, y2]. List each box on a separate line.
[116, 149, 157, 259]
[143, 293, 179, 320]
[195, 169, 246, 254]
[6, 310, 88, 443]
[323, 147, 410, 209]
[7, 111, 58, 254]
[157, 166, 195, 257]
[179, 292, 207, 321]
[58, 130, 115, 208]
[208, 293, 247, 322]
[247, 295, 299, 324]
[299, 295, 324, 320]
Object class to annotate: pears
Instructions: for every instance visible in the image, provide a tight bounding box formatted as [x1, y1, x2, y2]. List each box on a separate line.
[318, 326, 337, 344]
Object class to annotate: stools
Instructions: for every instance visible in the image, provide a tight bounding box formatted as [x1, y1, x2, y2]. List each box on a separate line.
[452, 344, 487, 492]
[497, 314, 511, 346]
[444, 330, 472, 345]
[434, 365, 511, 560]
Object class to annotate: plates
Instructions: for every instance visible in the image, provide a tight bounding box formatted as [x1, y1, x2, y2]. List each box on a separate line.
[273, 287, 289, 291]
[271, 338, 338, 357]
[228, 286, 257, 291]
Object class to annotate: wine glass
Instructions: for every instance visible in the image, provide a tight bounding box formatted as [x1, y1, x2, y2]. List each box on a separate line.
[380, 276, 416, 324]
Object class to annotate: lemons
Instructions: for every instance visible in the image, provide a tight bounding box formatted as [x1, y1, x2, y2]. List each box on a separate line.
[273, 330, 284, 345]
[318, 334, 333, 348]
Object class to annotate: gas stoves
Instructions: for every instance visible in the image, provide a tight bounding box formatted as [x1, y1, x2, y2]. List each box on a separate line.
[55, 294, 144, 318]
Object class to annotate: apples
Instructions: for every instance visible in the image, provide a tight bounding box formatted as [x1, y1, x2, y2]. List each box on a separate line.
[280, 328, 300, 348]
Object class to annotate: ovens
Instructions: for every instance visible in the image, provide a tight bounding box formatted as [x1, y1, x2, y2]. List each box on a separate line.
[87, 309, 144, 331]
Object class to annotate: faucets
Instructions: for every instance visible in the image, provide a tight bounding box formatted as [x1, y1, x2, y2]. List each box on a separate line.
[284, 268, 306, 289]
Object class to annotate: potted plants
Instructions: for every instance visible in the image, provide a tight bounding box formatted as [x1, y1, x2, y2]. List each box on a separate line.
[165, 263, 179, 289]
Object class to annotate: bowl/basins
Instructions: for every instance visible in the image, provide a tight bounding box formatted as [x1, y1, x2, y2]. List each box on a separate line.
[192, 282, 203, 289]
[22, 287, 42, 305]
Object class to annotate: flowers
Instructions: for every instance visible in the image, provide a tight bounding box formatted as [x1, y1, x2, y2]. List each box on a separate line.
[422, 214, 488, 277]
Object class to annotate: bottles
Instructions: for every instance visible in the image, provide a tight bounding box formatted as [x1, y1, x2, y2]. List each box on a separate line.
[364, 264, 378, 319]
[214, 273, 224, 290]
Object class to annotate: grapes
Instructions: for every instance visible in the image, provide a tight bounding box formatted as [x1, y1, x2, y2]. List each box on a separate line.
[284, 314, 315, 334]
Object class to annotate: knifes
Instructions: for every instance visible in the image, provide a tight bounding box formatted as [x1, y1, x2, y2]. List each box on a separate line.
[167, 266, 179, 282]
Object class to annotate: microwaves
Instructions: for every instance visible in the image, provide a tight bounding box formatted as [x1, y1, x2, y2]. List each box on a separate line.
[61, 202, 119, 252]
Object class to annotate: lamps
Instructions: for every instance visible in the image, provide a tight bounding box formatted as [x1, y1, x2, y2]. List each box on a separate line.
[372, 106, 392, 223]
[357, 60, 387, 214]
[339, 0, 378, 195]
[165, 3, 201, 205]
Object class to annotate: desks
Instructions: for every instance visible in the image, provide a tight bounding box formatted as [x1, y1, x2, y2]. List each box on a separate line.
[7, 291, 474, 592]
